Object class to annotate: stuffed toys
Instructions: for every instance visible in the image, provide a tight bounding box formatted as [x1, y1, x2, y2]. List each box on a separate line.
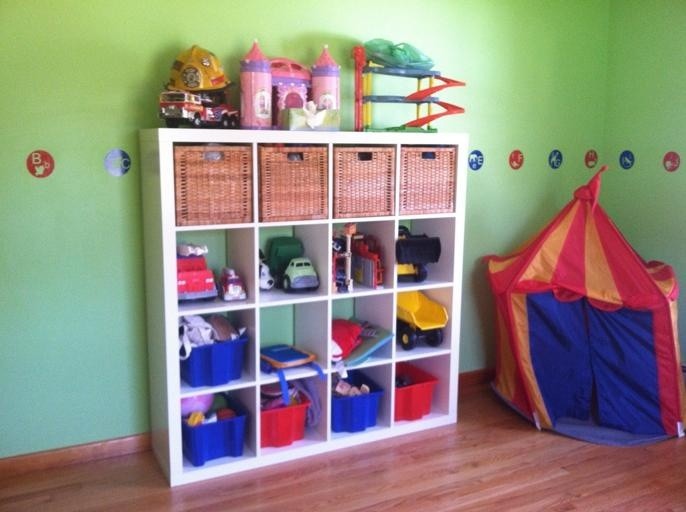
[259, 259, 274, 291]
[332, 318, 371, 362]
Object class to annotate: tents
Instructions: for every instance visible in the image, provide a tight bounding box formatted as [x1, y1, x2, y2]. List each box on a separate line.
[484, 164, 686, 441]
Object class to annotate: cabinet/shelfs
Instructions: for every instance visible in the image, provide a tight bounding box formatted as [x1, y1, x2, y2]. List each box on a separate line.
[138, 125, 470, 489]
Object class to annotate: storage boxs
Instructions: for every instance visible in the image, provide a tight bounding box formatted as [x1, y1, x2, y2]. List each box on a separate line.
[394, 361, 439, 423]
[331, 371, 384, 435]
[258, 389, 311, 448]
[180, 331, 250, 387]
[181, 399, 248, 467]
